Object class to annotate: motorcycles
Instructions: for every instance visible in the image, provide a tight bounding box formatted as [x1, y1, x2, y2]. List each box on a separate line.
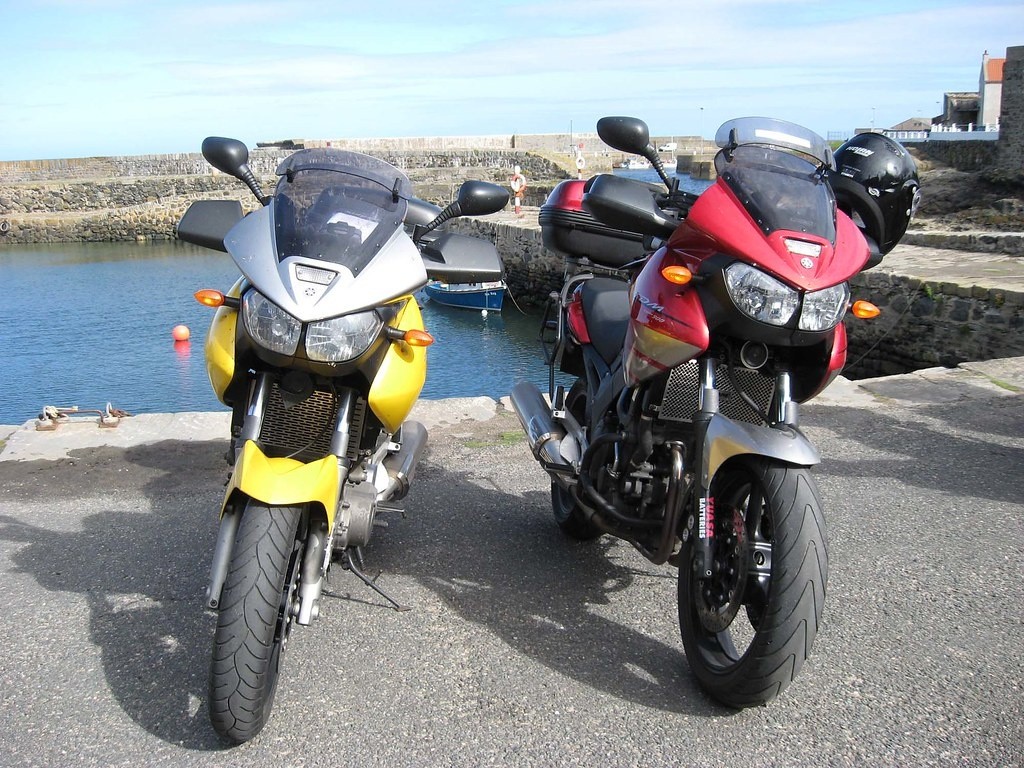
[193, 135, 511, 742]
[511, 114, 921, 707]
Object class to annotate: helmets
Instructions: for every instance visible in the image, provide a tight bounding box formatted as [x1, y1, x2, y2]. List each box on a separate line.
[824, 131, 919, 257]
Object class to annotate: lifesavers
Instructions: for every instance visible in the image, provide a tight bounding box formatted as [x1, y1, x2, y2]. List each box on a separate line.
[511, 174, 526, 193]
[575, 157, 586, 170]
[0, 221, 10, 232]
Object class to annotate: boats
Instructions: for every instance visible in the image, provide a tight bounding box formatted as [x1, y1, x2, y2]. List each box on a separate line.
[424, 278, 507, 312]
